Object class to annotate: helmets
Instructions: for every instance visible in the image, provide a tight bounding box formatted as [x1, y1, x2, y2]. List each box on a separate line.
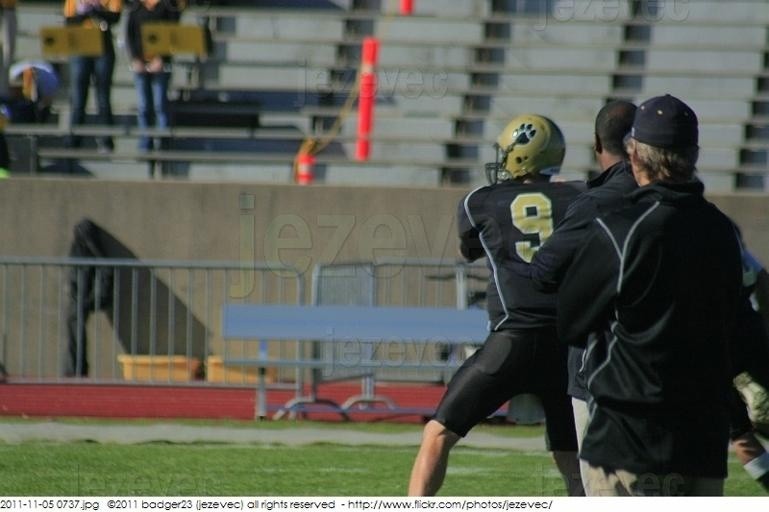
[495, 114, 566, 179]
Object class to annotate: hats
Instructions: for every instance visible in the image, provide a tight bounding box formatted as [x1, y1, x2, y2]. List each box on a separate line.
[630, 93, 698, 150]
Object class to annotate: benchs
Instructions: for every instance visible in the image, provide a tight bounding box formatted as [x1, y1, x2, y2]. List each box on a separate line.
[0, 1, 769, 189]
[221, 302, 510, 428]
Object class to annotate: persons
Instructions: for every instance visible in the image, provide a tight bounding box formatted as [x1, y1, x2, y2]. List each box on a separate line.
[55, 2, 119, 153]
[408, 93, 768, 496]
[61, 216, 104, 379]
[125, 1, 181, 158]
